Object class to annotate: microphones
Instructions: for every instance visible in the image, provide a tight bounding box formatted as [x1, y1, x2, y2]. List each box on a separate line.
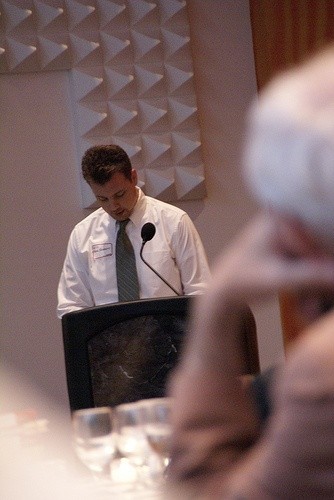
[140, 223, 180, 296]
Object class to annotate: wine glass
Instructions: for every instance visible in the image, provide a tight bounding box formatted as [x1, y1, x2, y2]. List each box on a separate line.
[0, 398, 173, 500]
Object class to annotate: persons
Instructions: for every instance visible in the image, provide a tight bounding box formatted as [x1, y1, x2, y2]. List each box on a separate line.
[56, 144, 213, 318]
[161, 47, 334, 500]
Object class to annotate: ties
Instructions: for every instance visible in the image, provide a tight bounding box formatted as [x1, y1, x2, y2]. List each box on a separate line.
[116, 218, 140, 300]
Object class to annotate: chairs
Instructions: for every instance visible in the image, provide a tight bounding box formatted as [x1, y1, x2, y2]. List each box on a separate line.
[61, 295, 260, 418]
